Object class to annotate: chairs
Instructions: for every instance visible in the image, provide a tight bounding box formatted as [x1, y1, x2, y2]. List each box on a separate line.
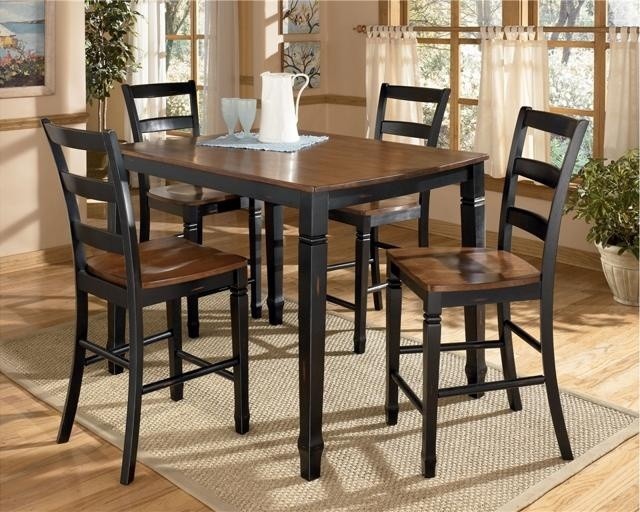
[124, 79, 262, 338]
[41, 117, 252, 485]
[327, 82, 452, 354]
[384, 107, 590, 478]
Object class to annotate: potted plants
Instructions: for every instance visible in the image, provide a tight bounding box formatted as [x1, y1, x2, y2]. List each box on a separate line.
[563, 146, 640, 306]
[85, 0, 150, 183]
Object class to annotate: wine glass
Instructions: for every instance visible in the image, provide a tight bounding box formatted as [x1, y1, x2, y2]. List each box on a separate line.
[220, 98, 256, 143]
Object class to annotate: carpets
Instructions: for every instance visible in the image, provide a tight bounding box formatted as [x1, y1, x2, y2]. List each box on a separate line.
[0, 287, 640, 512]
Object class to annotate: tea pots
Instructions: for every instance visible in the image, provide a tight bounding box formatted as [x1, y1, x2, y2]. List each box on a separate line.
[257, 72, 310, 144]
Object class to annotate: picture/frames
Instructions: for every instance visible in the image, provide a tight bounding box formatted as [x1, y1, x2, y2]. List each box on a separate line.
[0, 0, 57, 99]
